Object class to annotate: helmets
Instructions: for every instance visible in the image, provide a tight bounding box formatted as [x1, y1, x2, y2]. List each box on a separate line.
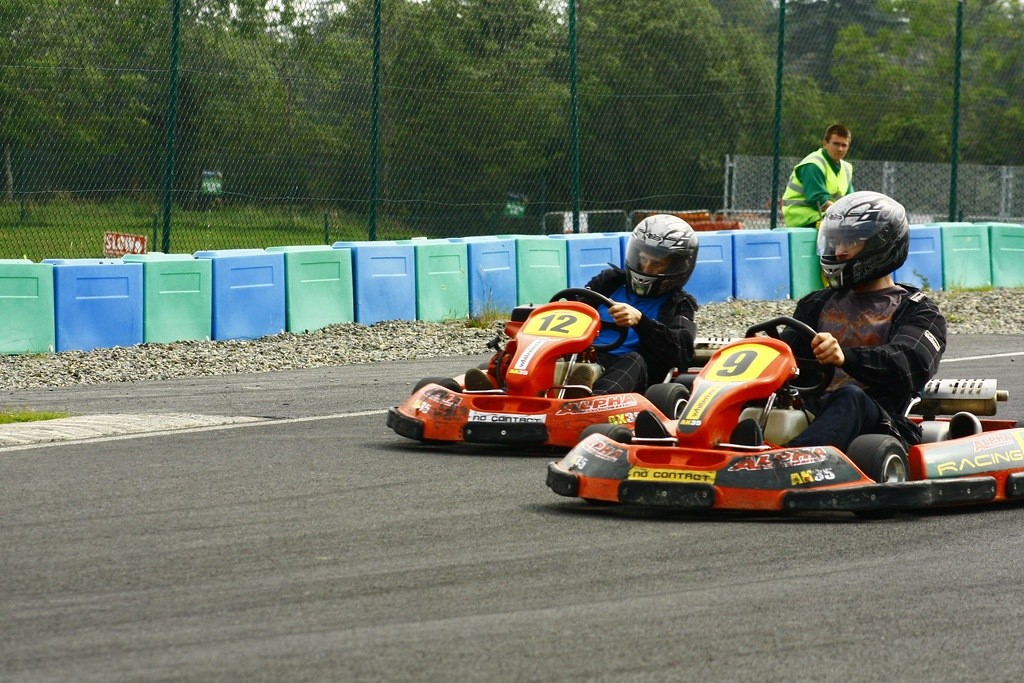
[625, 215, 699, 296]
[815, 189, 911, 292]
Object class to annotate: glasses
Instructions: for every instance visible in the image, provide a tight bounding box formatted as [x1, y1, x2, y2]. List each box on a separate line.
[827, 236, 864, 251]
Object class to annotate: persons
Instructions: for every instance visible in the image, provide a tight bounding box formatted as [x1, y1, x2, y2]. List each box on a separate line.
[780, 124, 856, 228]
[635, 190, 947, 451]
[463, 213, 701, 399]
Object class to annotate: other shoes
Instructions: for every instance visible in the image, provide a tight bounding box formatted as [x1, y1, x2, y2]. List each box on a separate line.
[729, 418, 764, 446]
[635, 409, 672, 445]
[564, 366, 593, 398]
[464, 368, 492, 391]
